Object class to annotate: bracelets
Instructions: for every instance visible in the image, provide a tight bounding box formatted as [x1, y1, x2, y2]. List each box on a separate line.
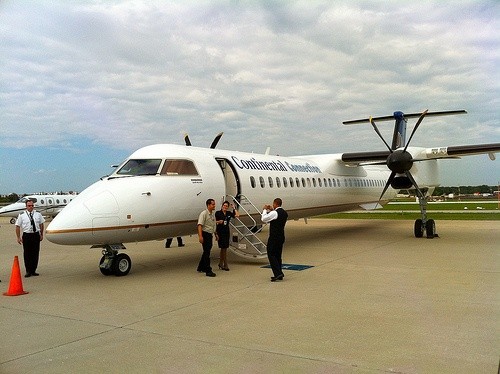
[214, 232, 217, 235]
[233, 209, 236, 210]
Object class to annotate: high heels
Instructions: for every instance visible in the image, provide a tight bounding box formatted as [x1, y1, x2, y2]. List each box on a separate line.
[219, 264, 224, 270]
[223, 266, 229, 271]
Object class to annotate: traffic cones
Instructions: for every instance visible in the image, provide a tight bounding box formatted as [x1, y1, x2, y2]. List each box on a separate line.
[4, 256, 29, 296]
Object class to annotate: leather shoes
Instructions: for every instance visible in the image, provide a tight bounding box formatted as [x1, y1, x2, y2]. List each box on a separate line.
[31, 272, 39, 276]
[25, 273, 31, 277]
[271, 273, 284, 281]
[270, 275, 283, 280]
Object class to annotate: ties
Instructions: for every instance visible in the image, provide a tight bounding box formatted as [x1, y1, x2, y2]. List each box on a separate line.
[30, 212, 36, 232]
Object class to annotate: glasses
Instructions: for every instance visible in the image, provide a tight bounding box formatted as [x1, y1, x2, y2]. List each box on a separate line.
[27, 205, 34, 207]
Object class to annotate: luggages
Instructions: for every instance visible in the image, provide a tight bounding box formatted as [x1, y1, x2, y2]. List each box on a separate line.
[206, 271, 216, 277]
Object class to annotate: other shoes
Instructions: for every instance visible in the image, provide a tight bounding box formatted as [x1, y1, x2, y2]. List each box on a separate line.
[166, 246, 170, 248]
[197, 268, 206, 272]
[178, 244, 184, 247]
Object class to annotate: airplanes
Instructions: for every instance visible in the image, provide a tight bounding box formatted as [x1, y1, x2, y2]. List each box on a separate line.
[46, 109, 500, 276]
[1, 195, 76, 225]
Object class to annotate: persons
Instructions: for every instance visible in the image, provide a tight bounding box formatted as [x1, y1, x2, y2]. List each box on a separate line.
[14, 200, 46, 278]
[165, 237, 185, 248]
[215, 200, 240, 271]
[261, 198, 288, 282]
[197, 198, 219, 277]
